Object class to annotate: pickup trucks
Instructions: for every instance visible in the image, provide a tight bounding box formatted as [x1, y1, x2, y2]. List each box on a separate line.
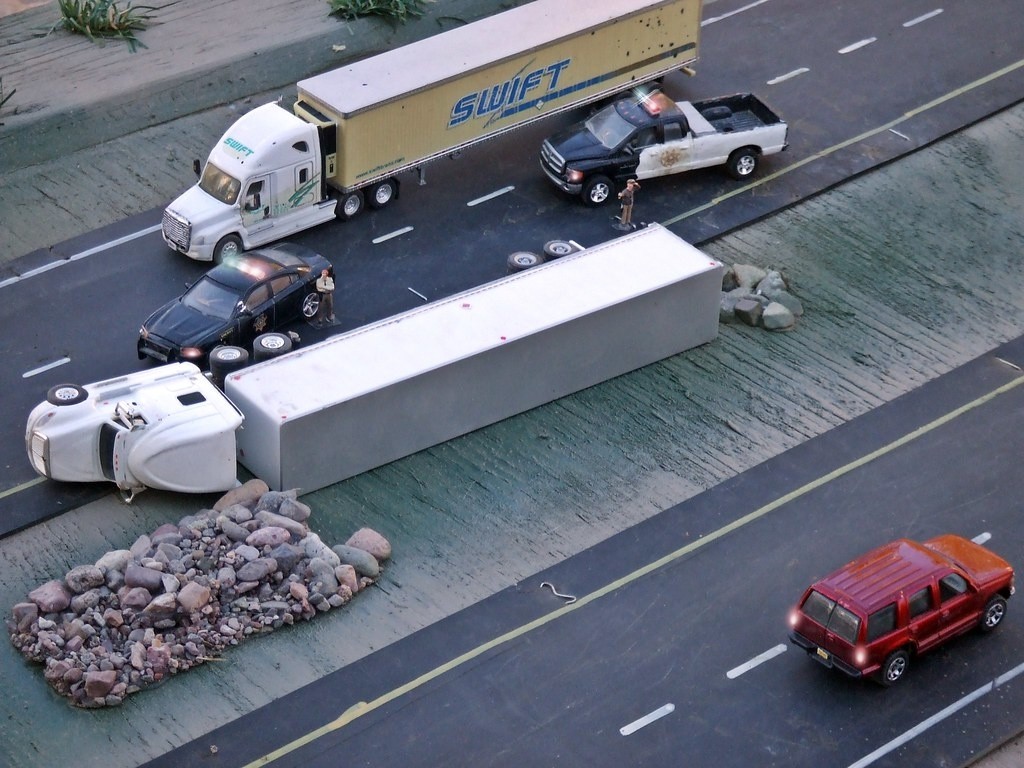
[538, 87, 789, 208]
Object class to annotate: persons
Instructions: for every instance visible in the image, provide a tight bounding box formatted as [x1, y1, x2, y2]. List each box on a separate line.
[316, 270, 335, 324]
[618, 179, 641, 227]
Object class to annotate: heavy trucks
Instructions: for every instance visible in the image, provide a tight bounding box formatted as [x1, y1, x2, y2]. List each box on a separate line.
[24, 219, 726, 504]
[161, 0, 702, 270]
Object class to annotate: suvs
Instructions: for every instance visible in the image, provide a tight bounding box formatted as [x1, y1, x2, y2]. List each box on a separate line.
[788, 533, 1014, 690]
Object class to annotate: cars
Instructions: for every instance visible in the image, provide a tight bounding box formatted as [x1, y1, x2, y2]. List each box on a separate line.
[137, 241, 335, 367]
